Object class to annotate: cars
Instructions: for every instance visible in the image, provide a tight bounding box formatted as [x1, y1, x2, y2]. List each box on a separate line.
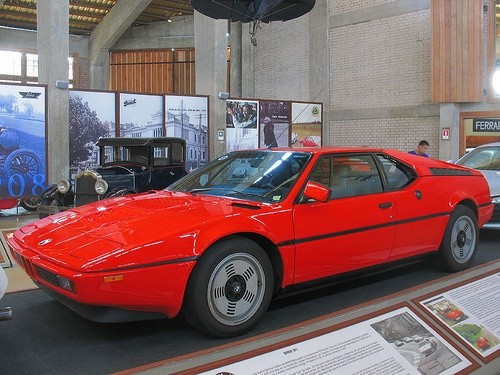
[291, 132, 298, 144]
[37, 136, 203, 220]
[299, 135, 321, 147]
[454, 142, 500, 230]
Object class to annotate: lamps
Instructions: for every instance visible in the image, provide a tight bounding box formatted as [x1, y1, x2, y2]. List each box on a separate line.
[54, 80, 69, 90]
[248, 20, 258, 47]
[218, 92, 230, 99]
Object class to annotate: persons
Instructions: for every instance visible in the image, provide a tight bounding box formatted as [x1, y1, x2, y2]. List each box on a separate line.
[299, 137, 318, 147]
[408, 140, 429, 157]
[263, 116, 278, 147]
[226, 102, 257, 128]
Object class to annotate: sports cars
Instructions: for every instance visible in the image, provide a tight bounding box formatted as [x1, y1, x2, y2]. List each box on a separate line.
[5, 146, 495, 341]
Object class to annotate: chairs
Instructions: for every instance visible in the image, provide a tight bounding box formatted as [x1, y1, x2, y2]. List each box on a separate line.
[271, 160, 292, 189]
